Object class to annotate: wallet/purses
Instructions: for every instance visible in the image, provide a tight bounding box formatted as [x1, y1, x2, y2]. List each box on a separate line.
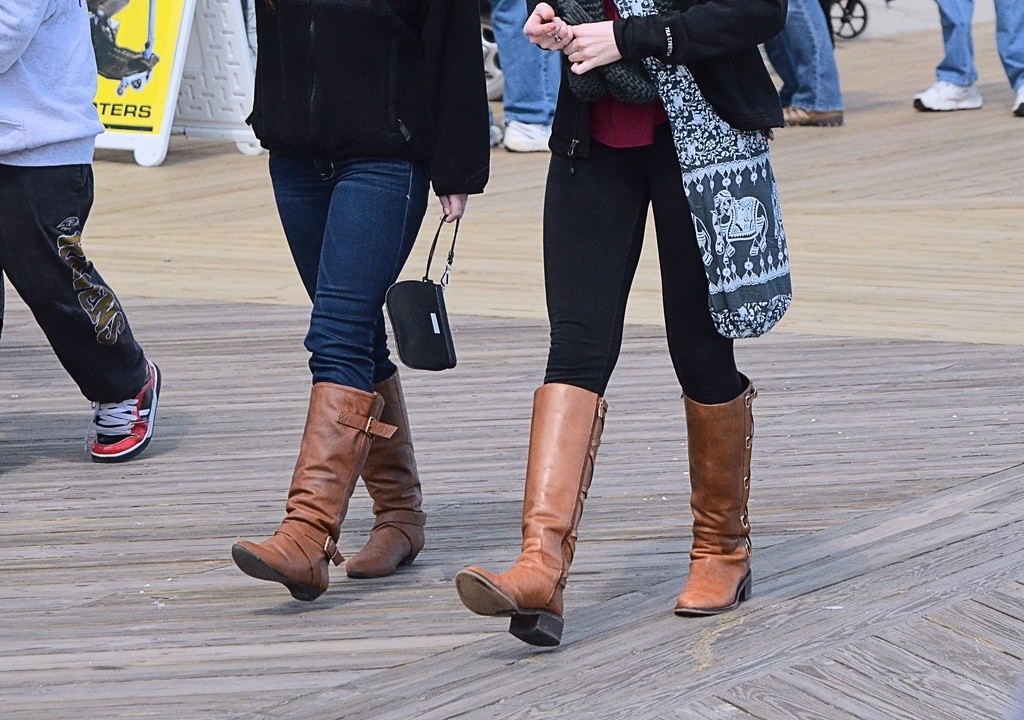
[385, 213, 460, 372]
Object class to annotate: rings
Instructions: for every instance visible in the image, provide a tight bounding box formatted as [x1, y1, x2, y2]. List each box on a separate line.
[554, 35, 562, 43]
[547, 33, 551, 37]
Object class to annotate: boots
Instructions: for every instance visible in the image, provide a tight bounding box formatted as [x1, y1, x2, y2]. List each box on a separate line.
[454, 381, 610, 649]
[230, 381, 399, 600]
[672, 371, 759, 617]
[344, 365, 427, 579]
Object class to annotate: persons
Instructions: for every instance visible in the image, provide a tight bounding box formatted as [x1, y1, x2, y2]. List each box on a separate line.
[457, 0, 843, 646]
[233, 0, 491, 602]
[914, 0, 1024, 117]
[0, 0, 160, 462]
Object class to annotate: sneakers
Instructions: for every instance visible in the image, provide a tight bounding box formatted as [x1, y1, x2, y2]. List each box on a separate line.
[489, 120, 551, 153]
[782, 106, 844, 128]
[1011, 86, 1024, 118]
[83, 358, 162, 463]
[912, 80, 984, 112]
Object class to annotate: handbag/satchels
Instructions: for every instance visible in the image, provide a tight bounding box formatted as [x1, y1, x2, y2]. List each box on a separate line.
[642, 49, 794, 341]
